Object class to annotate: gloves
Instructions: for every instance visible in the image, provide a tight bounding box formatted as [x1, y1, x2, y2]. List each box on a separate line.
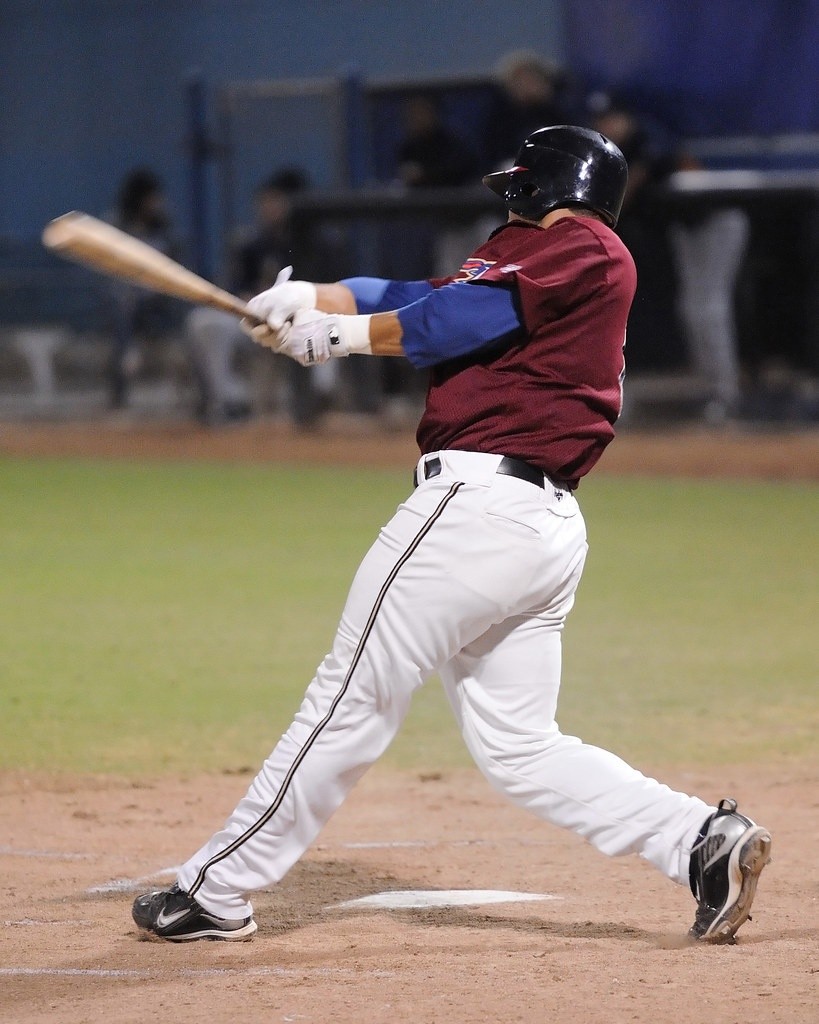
[239, 265, 319, 355]
[270, 308, 373, 368]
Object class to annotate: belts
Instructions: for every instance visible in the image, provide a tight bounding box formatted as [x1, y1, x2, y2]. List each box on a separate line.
[413, 455, 546, 491]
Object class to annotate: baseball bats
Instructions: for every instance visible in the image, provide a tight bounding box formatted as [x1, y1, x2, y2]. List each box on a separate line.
[42, 210, 266, 327]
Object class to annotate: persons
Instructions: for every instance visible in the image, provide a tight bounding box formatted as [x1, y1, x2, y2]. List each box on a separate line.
[479, 47, 585, 181]
[578, 99, 753, 429]
[220, 162, 390, 442]
[129, 120, 775, 948]
[95, 163, 220, 433]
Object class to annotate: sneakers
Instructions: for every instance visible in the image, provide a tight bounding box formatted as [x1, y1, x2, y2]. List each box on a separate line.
[689, 796, 773, 946]
[132, 880, 258, 943]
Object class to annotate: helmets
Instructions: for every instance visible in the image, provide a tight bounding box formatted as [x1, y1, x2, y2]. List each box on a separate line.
[480, 124, 629, 232]
